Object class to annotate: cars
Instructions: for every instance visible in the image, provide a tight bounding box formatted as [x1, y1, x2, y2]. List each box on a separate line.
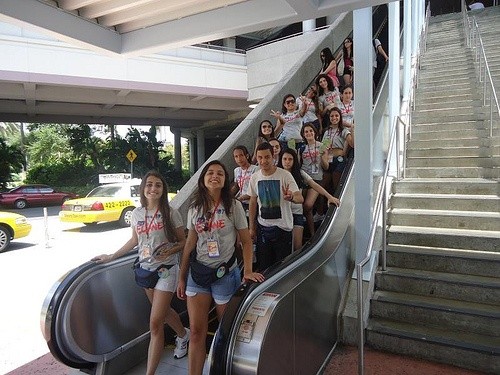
[0, 184, 81, 209]
[58, 178, 178, 228]
[0, 210, 33, 255]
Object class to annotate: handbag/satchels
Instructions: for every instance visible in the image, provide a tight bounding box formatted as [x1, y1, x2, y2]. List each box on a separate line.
[260, 226, 279, 244]
[336, 56, 344, 76]
[133, 264, 169, 289]
[188, 250, 236, 286]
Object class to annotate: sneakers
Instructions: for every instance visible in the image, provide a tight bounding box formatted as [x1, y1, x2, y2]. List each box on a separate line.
[172, 326, 190, 359]
[313, 211, 325, 222]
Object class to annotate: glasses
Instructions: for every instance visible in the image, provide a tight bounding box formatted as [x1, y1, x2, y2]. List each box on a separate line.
[286, 99, 295, 105]
[272, 145, 280, 149]
[320, 55, 326, 58]
[262, 124, 272, 129]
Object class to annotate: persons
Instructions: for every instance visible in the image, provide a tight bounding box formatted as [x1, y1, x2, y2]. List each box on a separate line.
[91, 169, 188, 375]
[176, 160, 265, 375]
[230, 37, 388, 284]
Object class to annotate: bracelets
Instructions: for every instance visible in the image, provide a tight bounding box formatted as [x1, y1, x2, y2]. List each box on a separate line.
[289, 196, 293, 201]
